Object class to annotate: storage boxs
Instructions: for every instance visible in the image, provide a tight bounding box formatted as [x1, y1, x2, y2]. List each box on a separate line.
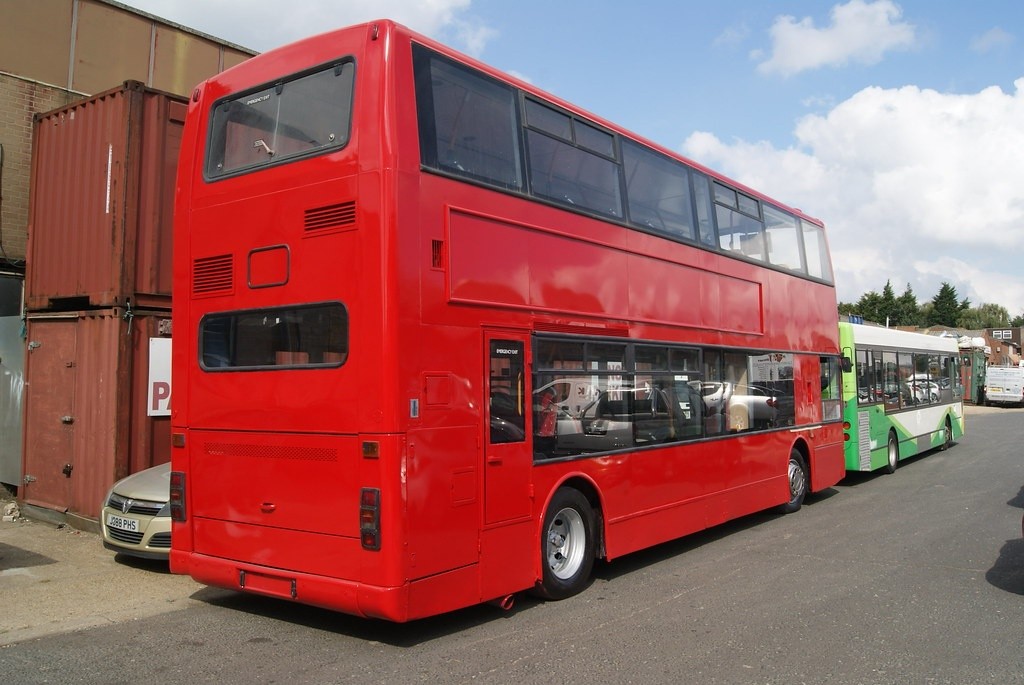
[23, 79, 314, 309]
[17, 307, 510, 520]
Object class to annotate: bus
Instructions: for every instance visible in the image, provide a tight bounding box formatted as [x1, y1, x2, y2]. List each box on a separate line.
[839, 322, 972, 474]
[166, 15, 856, 624]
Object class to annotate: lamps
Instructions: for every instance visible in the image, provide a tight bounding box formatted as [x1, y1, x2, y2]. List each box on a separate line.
[963, 356, 970, 365]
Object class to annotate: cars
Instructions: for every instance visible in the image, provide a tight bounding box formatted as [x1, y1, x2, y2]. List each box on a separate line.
[491, 376, 791, 459]
[97, 460, 171, 560]
[877, 374, 960, 410]
